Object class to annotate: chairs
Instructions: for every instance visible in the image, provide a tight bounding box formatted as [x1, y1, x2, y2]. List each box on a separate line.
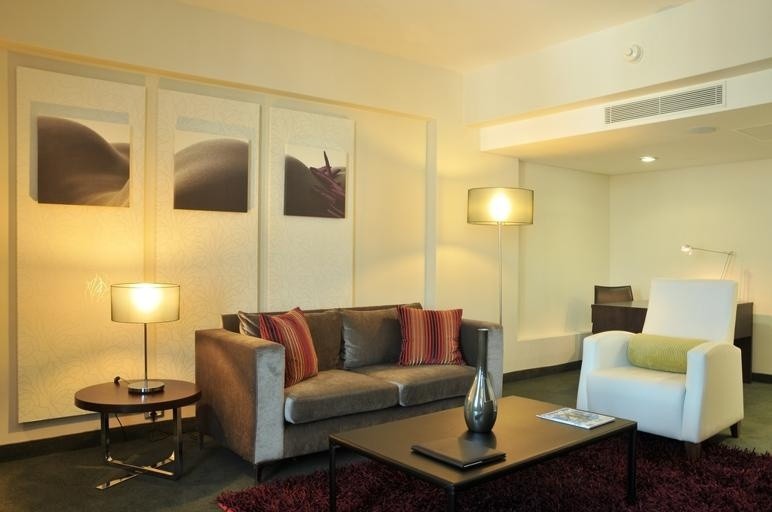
[592, 283, 634, 304]
[575, 278, 748, 469]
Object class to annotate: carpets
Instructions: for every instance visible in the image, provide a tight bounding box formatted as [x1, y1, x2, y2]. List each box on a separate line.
[211, 429, 772, 511]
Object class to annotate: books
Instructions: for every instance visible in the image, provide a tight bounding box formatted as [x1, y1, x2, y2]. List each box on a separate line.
[537, 408, 614, 429]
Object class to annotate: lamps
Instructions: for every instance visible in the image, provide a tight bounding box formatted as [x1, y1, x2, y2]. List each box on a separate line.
[110, 281, 181, 396]
[467, 187, 535, 331]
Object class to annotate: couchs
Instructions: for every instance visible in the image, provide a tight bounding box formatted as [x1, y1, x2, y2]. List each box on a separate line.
[194, 302, 506, 484]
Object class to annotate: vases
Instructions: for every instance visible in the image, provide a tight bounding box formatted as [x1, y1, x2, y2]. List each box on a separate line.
[461, 327, 498, 434]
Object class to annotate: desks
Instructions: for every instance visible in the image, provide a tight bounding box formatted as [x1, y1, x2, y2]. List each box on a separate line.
[74, 378, 203, 490]
[589, 300, 757, 382]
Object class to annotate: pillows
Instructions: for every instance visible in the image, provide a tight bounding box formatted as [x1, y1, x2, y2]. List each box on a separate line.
[236, 309, 345, 373]
[339, 301, 424, 371]
[396, 304, 468, 368]
[628, 331, 709, 376]
[257, 307, 319, 388]
